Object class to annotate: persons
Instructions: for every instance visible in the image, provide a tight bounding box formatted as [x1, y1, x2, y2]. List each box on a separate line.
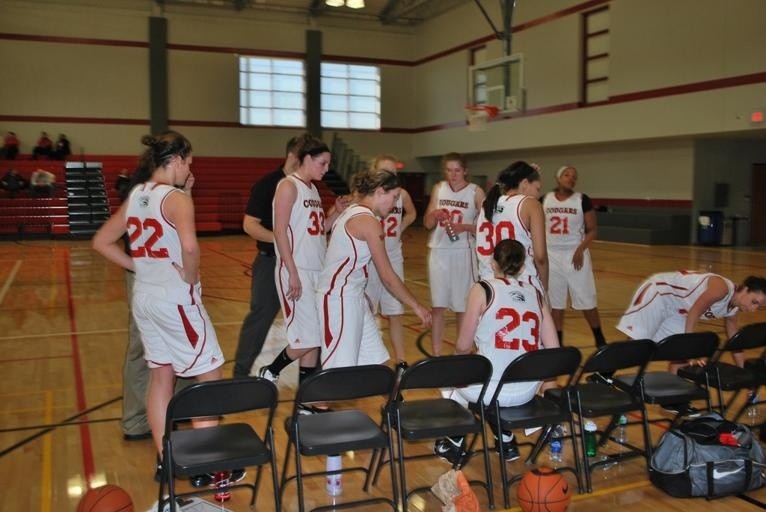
[475, 161, 559, 349]
[1, 127, 70, 197]
[311, 169, 431, 409]
[326, 154, 416, 375]
[254, 140, 335, 416]
[114, 157, 153, 205]
[614, 268, 766, 419]
[119, 171, 226, 440]
[234, 132, 314, 377]
[423, 153, 488, 356]
[537, 165, 606, 349]
[430, 237, 560, 469]
[90, 129, 248, 488]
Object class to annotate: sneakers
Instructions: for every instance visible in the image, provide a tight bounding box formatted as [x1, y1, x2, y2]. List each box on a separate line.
[298, 404, 331, 416]
[661, 401, 702, 418]
[192, 469, 246, 487]
[257, 366, 279, 386]
[494, 432, 520, 462]
[433, 436, 465, 467]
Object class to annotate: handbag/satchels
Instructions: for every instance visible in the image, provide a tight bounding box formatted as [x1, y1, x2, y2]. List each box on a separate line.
[650, 411, 766, 499]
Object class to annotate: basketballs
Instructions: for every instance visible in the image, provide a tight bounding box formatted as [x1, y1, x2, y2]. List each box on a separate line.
[518, 466, 572, 511]
[76, 484, 134, 512]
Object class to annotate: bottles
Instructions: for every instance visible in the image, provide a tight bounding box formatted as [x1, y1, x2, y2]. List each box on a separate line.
[749, 409, 760, 441]
[215, 471, 230, 501]
[445, 218, 459, 242]
[550, 426, 564, 463]
[326, 456, 343, 496]
[745, 388, 759, 417]
[585, 420, 597, 461]
[601, 454, 612, 488]
[616, 416, 629, 443]
[617, 455, 626, 482]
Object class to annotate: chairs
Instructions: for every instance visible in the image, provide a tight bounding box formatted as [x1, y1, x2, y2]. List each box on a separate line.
[677, 323, 766, 422]
[458, 346, 584, 509]
[604, 331, 721, 469]
[532, 338, 657, 493]
[159, 376, 280, 512]
[371, 353, 496, 512]
[280, 364, 400, 512]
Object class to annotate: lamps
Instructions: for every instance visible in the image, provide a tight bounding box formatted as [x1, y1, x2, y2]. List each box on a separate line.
[325, 0, 366, 9]
